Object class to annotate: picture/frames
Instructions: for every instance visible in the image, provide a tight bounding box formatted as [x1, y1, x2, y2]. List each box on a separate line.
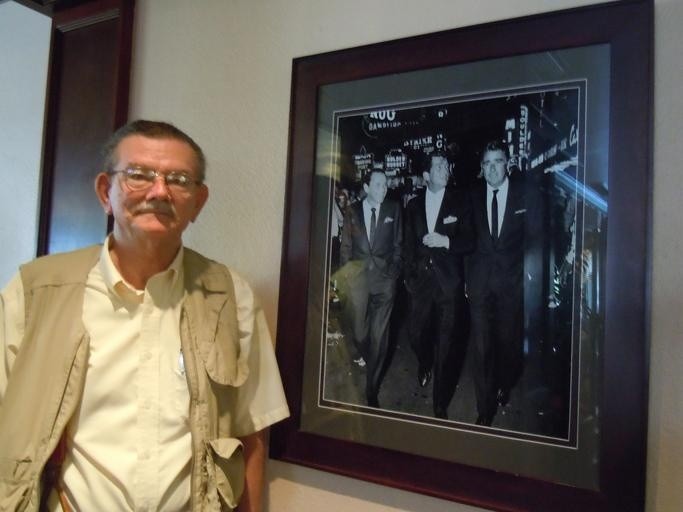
[267, 0, 654, 511]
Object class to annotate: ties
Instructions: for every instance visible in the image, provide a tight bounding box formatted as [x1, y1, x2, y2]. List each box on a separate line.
[490, 189, 500, 245]
[369, 208, 377, 251]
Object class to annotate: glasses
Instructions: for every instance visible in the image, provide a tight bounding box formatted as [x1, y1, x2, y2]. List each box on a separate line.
[109, 164, 201, 193]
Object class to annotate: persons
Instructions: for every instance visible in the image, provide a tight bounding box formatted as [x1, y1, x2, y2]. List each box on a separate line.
[0, 117, 292, 511]
[337, 171, 402, 409]
[402, 149, 468, 425]
[468, 141, 551, 429]
[329, 165, 422, 301]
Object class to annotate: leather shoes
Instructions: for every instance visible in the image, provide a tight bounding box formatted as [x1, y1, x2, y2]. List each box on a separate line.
[433, 404, 449, 420]
[498, 384, 510, 408]
[475, 415, 494, 426]
[417, 368, 431, 388]
[366, 387, 380, 408]
